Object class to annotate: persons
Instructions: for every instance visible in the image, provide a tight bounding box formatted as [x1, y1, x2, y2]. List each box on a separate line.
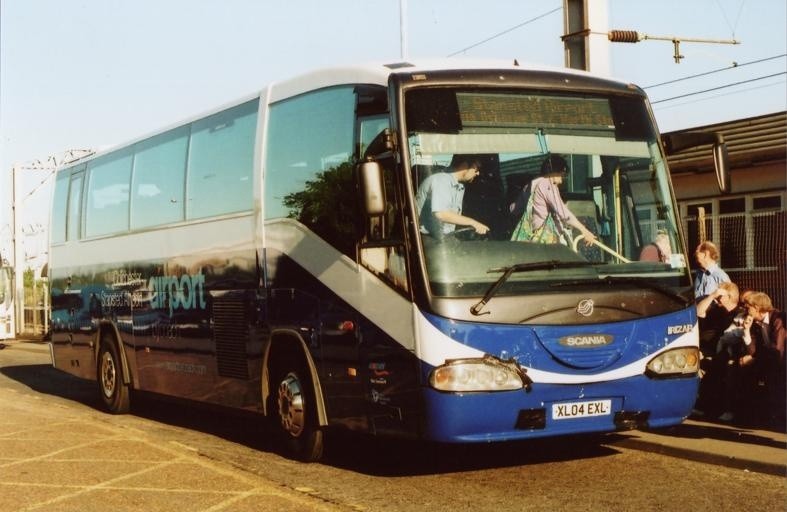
[639, 224, 671, 262]
[341, 321, 391, 379]
[693, 240, 787, 427]
[417, 154, 492, 234]
[510, 153, 597, 248]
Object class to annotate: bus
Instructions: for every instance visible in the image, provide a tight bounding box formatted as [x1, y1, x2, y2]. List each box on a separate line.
[0, 255, 15, 349]
[41, 63, 700, 462]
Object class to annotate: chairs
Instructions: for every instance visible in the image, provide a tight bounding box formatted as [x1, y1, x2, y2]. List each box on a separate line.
[573, 214, 604, 265]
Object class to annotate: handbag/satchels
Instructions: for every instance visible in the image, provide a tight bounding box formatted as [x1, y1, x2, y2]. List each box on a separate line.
[509, 182, 557, 244]
